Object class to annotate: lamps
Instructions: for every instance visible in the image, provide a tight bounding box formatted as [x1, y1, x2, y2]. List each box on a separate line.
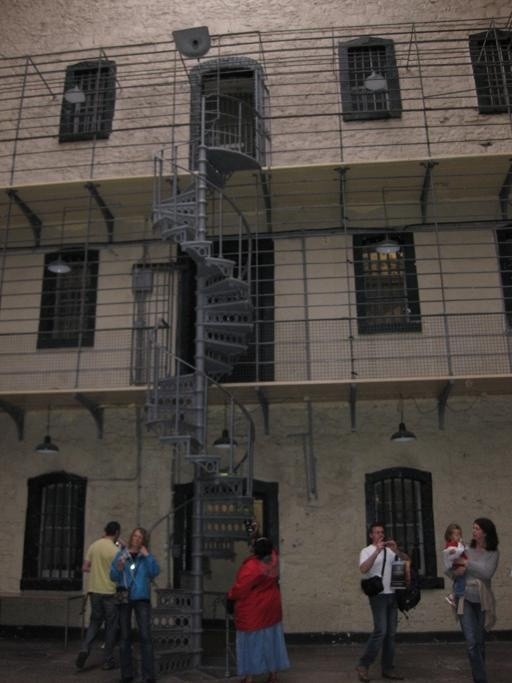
[64, 83, 86, 103]
[34, 399, 59, 453]
[213, 396, 238, 449]
[389, 394, 417, 442]
[47, 207, 72, 274]
[364, 67, 386, 91]
[375, 188, 401, 253]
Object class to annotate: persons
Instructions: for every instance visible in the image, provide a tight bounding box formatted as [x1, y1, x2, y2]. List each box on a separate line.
[230, 535, 291, 682]
[110, 526, 161, 683]
[75, 520, 123, 670]
[439, 522, 466, 606]
[355, 522, 412, 682]
[450, 517, 500, 682]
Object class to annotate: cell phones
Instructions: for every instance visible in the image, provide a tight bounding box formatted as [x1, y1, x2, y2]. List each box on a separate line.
[115, 542, 119, 545]
[245, 518, 252, 530]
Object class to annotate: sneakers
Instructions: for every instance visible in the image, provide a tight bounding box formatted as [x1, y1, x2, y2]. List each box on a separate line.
[446, 594, 456, 608]
[103, 661, 115, 670]
[357, 666, 369, 681]
[383, 670, 403, 680]
[76, 651, 88, 668]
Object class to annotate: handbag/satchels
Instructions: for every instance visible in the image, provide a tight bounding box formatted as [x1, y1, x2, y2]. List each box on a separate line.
[114, 591, 129, 604]
[395, 555, 420, 611]
[361, 576, 384, 594]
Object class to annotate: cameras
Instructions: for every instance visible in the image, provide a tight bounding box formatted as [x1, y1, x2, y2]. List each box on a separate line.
[382, 536, 390, 542]
[121, 551, 131, 560]
